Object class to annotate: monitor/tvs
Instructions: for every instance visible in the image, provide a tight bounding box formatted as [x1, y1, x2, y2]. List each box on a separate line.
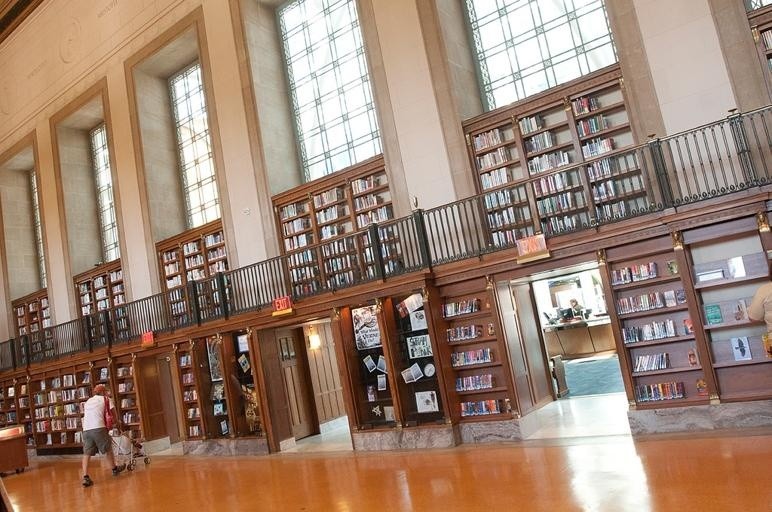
[561, 309, 574, 319]
[543, 312, 551, 321]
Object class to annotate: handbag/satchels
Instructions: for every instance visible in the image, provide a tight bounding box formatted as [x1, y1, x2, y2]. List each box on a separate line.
[103, 395, 117, 430]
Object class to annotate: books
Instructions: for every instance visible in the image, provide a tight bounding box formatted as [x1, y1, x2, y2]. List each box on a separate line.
[732, 299, 747, 322]
[443, 298, 512, 417]
[473, 97, 627, 248]
[78, 270, 130, 348]
[703, 305, 723, 324]
[762, 333, 772, 358]
[610, 259, 710, 403]
[761, 29, 772, 74]
[163, 232, 232, 328]
[731, 336, 752, 361]
[18, 298, 55, 364]
[728, 256, 746, 278]
[0, 367, 135, 446]
[697, 269, 724, 276]
[179, 355, 203, 437]
[280, 175, 401, 298]
[699, 272, 724, 281]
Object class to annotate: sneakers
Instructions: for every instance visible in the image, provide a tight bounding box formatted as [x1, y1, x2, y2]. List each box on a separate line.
[112, 462, 126, 476]
[82, 476, 94, 486]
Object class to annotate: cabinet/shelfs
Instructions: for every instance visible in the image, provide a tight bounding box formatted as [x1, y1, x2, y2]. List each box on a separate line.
[0, 356, 147, 456]
[72, 257, 131, 350]
[10, 287, 56, 367]
[460, 63, 666, 250]
[270, 152, 404, 303]
[153, 217, 233, 329]
[598, 213, 772, 414]
[171, 326, 265, 443]
[339, 288, 519, 443]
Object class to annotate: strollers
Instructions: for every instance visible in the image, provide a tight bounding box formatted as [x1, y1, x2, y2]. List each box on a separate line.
[109, 429, 151, 476]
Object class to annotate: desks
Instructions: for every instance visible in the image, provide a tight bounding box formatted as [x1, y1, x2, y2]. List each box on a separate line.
[0, 431, 32, 477]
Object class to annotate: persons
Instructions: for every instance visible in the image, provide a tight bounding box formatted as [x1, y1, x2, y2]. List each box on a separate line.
[747, 262, 772, 356]
[570, 299, 583, 311]
[82, 385, 126, 485]
